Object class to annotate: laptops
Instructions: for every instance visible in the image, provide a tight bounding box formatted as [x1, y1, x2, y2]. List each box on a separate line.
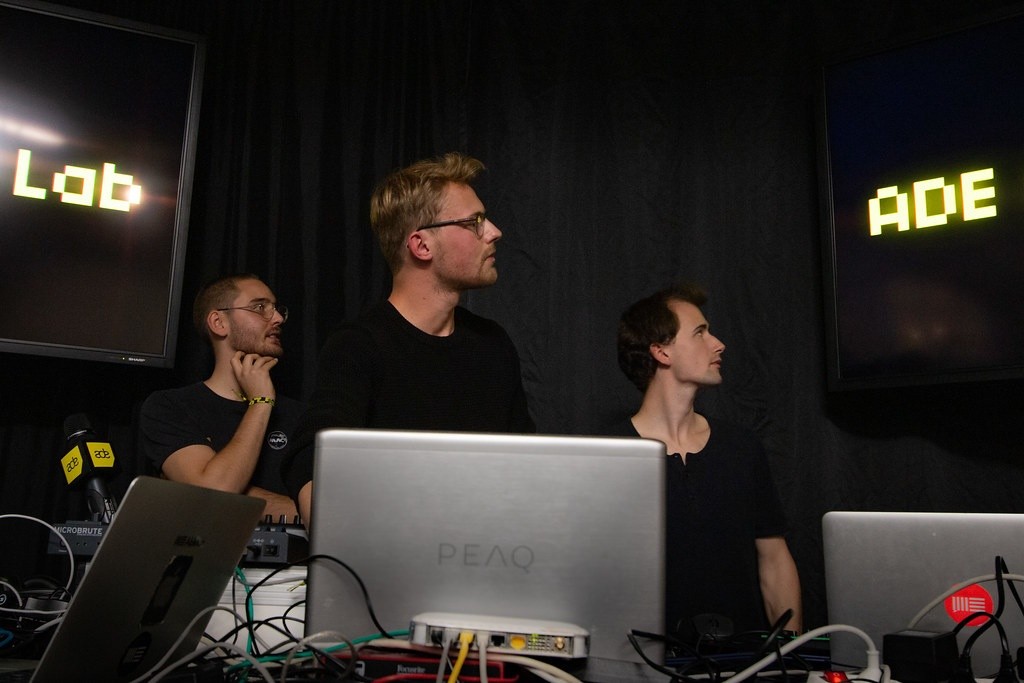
[0, 477, 268, 683]
[304, 428, 665, 683]
[823, 511, 1024, 682]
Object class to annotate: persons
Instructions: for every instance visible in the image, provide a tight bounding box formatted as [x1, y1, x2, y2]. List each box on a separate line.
[137, 261, 304, 528]
[598, 286, 800, 647]
[279, 151, 535, 540]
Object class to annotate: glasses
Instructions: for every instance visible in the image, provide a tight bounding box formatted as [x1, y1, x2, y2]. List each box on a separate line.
[218, 302, 287, 323]
[417, 215, 485, 237]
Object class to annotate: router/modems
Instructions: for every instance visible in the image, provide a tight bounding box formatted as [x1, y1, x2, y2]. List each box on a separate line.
[409, 612, 588, 659]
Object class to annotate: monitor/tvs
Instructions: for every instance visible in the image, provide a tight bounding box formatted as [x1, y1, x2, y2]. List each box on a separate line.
[0, 0, 207, 368]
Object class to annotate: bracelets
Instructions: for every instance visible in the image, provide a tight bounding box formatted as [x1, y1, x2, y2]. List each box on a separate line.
[247, 395, 275, 405]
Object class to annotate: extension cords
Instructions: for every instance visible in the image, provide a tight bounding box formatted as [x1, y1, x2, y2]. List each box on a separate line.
[807, 671, 997, 683]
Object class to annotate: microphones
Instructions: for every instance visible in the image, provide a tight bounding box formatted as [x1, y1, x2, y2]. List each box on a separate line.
[60, 414, 119, 523]
